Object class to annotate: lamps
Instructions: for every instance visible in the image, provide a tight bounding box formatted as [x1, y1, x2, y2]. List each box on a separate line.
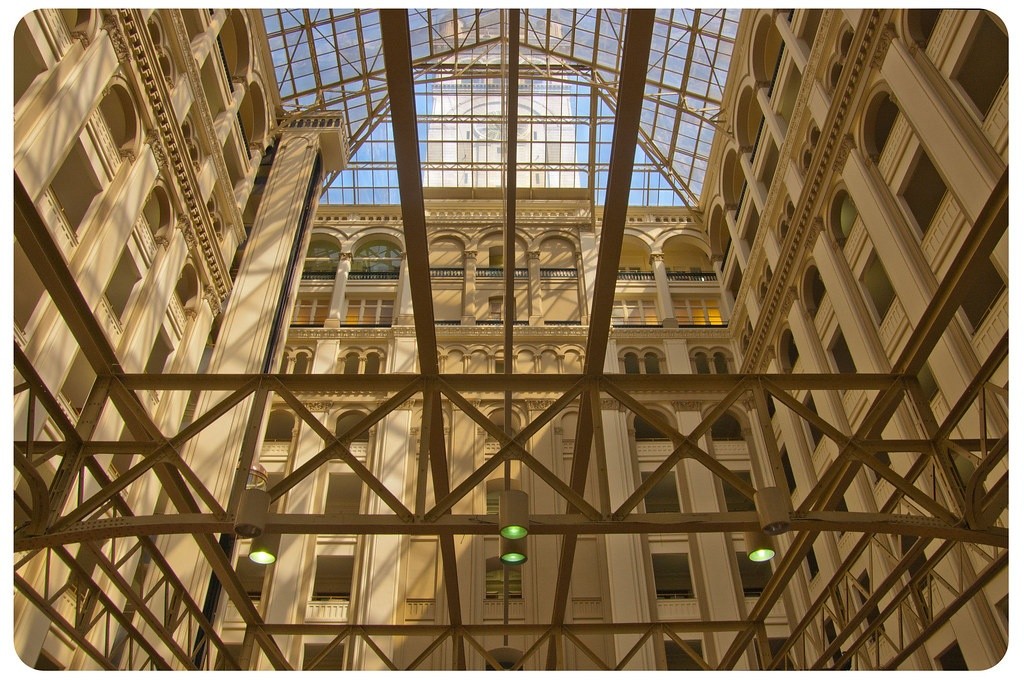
[500, 535, 527, 562]
[753, 486, 793, 534]
[234, 487, 270, 540]
[498, 490, 530, 542]
[745, 533, 776, 561]
[249, 534, 279, 565]
